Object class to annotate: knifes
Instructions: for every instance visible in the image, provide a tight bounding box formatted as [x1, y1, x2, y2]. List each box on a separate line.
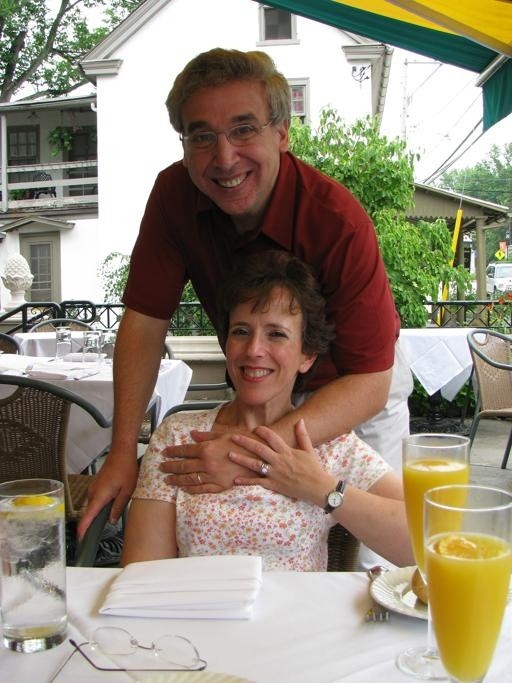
[23, 365, 33, 374]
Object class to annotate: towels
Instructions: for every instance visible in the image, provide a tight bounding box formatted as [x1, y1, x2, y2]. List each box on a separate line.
[19, 351, 107, 379]
[93, 549, 265, 625]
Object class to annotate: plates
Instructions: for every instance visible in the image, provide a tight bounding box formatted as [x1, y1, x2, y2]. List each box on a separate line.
[368, 564, 430, 621]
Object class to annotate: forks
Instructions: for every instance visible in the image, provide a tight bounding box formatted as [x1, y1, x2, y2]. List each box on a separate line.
[367, 564, 391, 625]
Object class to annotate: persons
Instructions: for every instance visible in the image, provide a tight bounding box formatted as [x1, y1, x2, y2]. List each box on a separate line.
[116, 246, 416, 572]
[75, 46, 412, 571]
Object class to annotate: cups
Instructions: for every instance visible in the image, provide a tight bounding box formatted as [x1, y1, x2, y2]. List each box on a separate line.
[82, 330, 103, 368]
[0, 476, 68, 653]
[56, 326, 72, 361]
[420, 484, 512, 683]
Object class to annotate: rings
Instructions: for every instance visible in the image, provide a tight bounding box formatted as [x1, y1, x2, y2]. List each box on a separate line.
[257, 461, 272, 471]
[193, 471, 204, 485]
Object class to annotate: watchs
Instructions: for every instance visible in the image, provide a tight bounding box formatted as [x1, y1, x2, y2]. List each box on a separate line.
[321, 479, 347, 516]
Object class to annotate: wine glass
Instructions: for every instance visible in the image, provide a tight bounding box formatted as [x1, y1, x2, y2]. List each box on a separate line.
[395, 429, 470, 681]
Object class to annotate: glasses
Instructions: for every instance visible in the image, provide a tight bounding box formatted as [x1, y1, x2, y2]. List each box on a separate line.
[178, 118, 274, 153]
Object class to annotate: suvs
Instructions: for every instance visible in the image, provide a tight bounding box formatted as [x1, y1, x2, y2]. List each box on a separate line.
[484, 260, 512, 297]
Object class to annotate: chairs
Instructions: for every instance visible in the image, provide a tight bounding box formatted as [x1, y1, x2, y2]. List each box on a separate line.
[0, 330, 25, 355]
[462, 328, 512, 470]
[137, 341, 176, 445]
[0, 374, 133, 555]
[28, 318, 97, 333]
[73, 452, 364, 570]
[31, 170, 57, 199]
